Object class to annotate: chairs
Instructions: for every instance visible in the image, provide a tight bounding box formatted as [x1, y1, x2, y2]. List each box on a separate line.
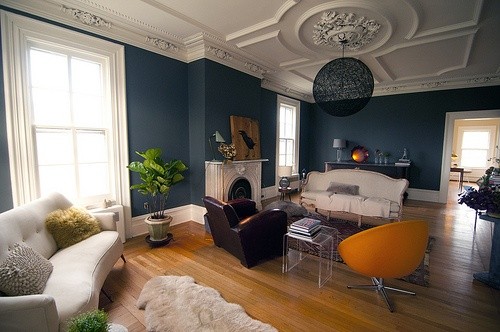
[337, 220, 430, 312]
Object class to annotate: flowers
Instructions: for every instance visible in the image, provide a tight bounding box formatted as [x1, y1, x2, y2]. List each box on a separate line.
[456, 186, 500, 213]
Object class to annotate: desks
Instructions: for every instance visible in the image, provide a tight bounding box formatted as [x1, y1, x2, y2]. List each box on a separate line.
[472, 213, 500, 290]
[450, 167, 464, 192]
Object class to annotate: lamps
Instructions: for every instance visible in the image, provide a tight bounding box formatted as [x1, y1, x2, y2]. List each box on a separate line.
[278, 166, 291, 189]
[333, 139, 346, 162]
[312, 39, 374, 118]
[208, 131, 227, 162]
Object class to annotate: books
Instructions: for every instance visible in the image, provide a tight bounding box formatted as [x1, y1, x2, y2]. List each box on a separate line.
[288, 217, 322, 242]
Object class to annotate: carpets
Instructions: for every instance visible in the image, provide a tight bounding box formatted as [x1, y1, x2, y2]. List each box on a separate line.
[288, 213, 438, 288]
[135, 274, 277, 332]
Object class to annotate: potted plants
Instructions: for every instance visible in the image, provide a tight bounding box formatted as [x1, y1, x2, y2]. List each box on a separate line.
[125, 146, 188, 246]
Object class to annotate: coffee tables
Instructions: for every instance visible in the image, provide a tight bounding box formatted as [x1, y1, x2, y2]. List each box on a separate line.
[282, 226, 338, 288]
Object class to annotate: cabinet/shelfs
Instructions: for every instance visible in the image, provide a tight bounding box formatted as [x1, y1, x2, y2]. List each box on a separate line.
[324, 162, 410, 204]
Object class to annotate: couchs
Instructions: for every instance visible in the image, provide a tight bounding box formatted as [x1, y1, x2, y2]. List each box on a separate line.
[0, 192, 127, 332]
[202, 195, 288, 269]
[299, 168, 409, 220]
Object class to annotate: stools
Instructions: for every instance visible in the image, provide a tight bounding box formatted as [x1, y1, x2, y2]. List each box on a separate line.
[278, 187, 292, 201]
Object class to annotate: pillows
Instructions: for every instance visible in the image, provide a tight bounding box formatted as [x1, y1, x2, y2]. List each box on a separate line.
[45, 204, 103, 249]
[0, 240, 54, 295]
